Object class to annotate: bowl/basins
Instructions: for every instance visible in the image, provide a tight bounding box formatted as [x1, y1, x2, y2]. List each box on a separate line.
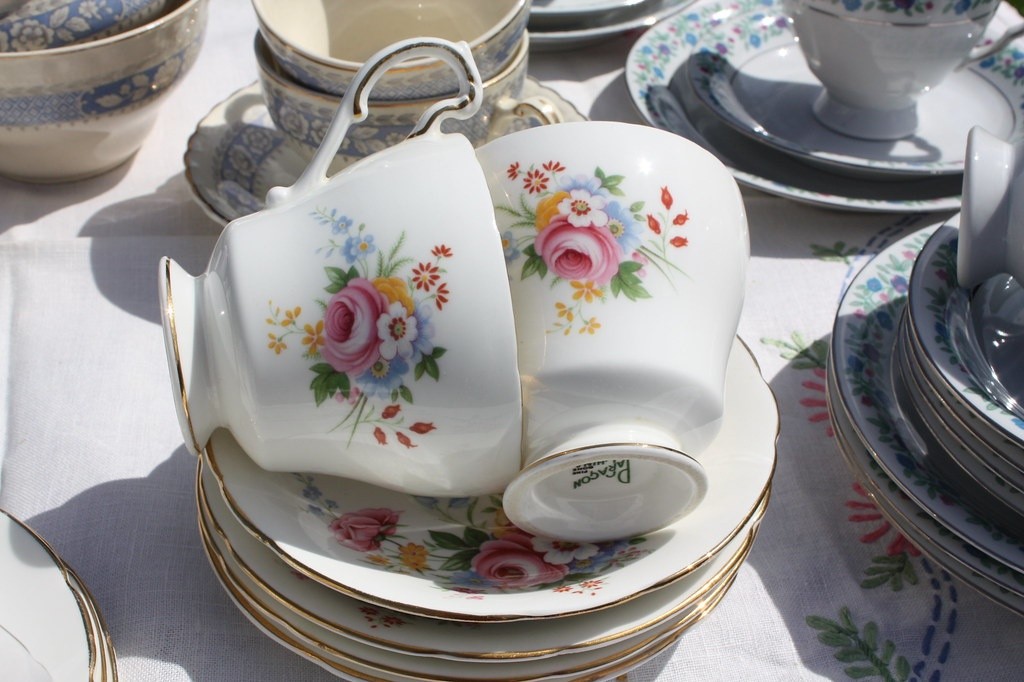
[249, 28, 532, 176]
[251, 1, 531, 100]
[1, 0, 171, 53]
[0, 2, 209, 184]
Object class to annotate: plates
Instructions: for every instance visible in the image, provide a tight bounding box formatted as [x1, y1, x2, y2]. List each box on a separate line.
[530, 4, 1021, 209]
[825, 207, 1024, 614]
[1, 507, 122, 682]
[193, 338, 782, 680]
[182, 76, 588, 231]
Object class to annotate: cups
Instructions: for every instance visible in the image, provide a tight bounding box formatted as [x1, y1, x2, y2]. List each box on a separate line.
[158, 37, 524, 503]
[787, 3, 1002, 141]
[477, 120, 749, 542]
